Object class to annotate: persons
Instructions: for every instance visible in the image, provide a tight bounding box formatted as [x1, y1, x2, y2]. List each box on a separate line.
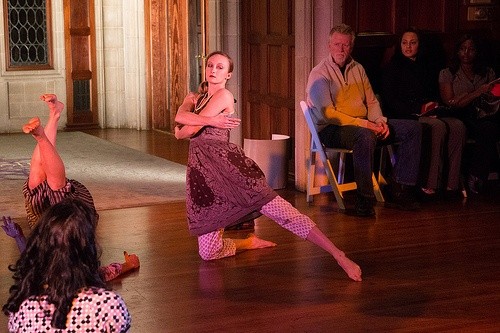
[1, 93, 139, 284]
[438, 30, 499, 195]
[174, 49, 362, 283]
[381, 26, 467, 204]
[2, 199, 131, 333]
[307, 23, 428, 217]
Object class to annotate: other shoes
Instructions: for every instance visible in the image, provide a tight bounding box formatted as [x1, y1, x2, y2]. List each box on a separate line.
[438, 188, 466, 202]
[418, 187, 442, 202]
[383, 198, 421, 212]
[346, 204, 377, 218]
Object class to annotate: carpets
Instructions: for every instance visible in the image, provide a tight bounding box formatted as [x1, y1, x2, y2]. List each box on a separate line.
[0, 131, 186, 218]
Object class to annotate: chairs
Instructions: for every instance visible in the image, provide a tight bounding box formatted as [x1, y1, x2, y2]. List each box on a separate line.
[300, 99, 387, 210]
